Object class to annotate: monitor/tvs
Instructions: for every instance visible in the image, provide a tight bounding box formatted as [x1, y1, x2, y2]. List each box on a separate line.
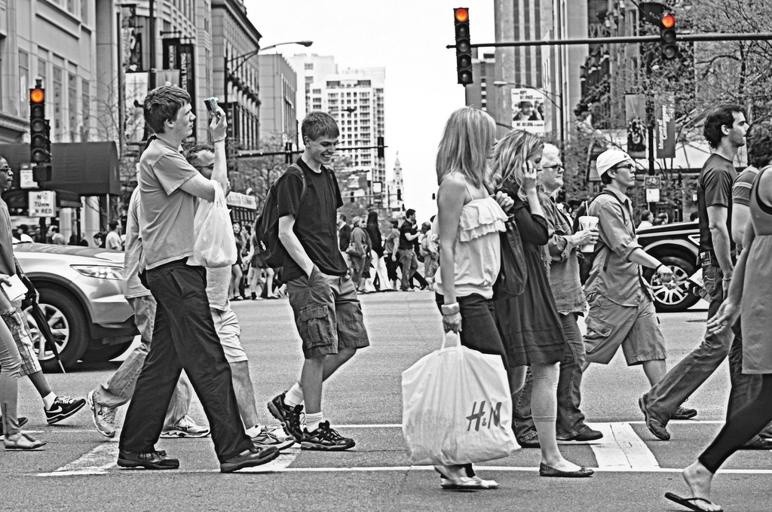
[205, 98, 219, 116]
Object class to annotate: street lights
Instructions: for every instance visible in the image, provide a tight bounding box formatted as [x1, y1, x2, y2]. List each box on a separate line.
[630, 114, 660, 220]
[325, 105, 358, 117]
[494, 80, 568, 198]
[221, 38, 314, 188]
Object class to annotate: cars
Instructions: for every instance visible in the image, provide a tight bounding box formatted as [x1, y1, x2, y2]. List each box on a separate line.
[635, 213, 705, 313]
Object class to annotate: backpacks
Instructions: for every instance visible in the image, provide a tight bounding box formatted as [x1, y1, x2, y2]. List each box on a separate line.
[250, 162, 337, 268]
[572, 190, 625, 285]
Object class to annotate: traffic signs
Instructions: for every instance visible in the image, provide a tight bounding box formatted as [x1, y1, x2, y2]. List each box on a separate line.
[27, 189, 55, 218]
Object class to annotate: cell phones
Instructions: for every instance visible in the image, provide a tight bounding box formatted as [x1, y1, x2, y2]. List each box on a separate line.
[517, 161, 536, 177]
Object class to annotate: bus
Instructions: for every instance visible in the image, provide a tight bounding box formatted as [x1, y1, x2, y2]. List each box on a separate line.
[218, 190, 260, 234]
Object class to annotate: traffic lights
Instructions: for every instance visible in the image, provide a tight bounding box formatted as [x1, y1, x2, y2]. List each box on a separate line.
[28, 88, 49, 163]
[660, 11, 678, 60]
[454, 8, 473, 85]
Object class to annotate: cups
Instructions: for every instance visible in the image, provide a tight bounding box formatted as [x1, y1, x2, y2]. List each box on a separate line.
[580, 216, 598, 255]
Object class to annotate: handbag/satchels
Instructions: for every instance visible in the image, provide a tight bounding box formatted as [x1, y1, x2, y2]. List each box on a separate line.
[401, 329, 522, 467]
[345, 242, 369, 258]
[482, 179, 528, 300]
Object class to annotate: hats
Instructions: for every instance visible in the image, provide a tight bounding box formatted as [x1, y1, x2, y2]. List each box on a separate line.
[595, 148, 632, 177]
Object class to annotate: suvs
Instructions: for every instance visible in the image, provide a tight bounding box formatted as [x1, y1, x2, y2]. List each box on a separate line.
[6, 239, 144, 375]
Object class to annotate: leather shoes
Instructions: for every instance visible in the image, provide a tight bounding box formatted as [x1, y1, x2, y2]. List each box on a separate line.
[519, 435, 541, 448]
[539, 462, 594, 477]
[557, 427, 603, 441]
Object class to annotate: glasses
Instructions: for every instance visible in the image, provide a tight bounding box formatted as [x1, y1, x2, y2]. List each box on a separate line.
[616, 164, 634, 169]
[543, 162, 566, 171]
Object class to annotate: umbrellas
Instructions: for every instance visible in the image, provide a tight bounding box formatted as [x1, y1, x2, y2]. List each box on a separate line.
[15, 262, 66, 376]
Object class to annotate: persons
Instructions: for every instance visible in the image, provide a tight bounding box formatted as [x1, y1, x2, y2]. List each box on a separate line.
[229, 221, 286, 300]
[515, 142, 603, 449]
[731, 117, 772, 439]
[15, 211, 126, 251]
[428, 108, 515, 492]
[640, 106, 772, 440]
[265, 111, 371, 453]
[664, 120, 772, 512]
[335, 209, 443, 294]
[88, 136, 213, 440]
[491, 130, 594, 477]
[179, 141, 296, 462]
[690, 211, 699, 222]
[637, 210, 669, 228]
[0, 273, 48, 451]
[582, 148, 699, 420]
[0, 156, 86, 435]
[116, 83, 280, 473]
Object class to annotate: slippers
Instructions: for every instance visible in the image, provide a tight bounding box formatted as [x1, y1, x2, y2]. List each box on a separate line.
[665, 492, 723, 512]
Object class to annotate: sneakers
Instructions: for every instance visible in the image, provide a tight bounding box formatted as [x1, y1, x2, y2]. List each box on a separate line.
[0, 416, 28, 436]
[639, 392, 670, 440]
[220, 444, 280, 473]
[433, 464, 499, 487]
[87, 389, 117, 438]
[300, 420, 355, 451]
[266, 390, 306, 443]
[737, 436, 772, 451]
[159, 414, 210, 438]
[229, 289, 288, 303]
[3, 433, 46, 449]
[117, 448, 179, 470]
[43, 396, 86, 425]
[246, 421, 295, 450]
[670, 406, 697, 420]
[353, 283, 434, 294]
